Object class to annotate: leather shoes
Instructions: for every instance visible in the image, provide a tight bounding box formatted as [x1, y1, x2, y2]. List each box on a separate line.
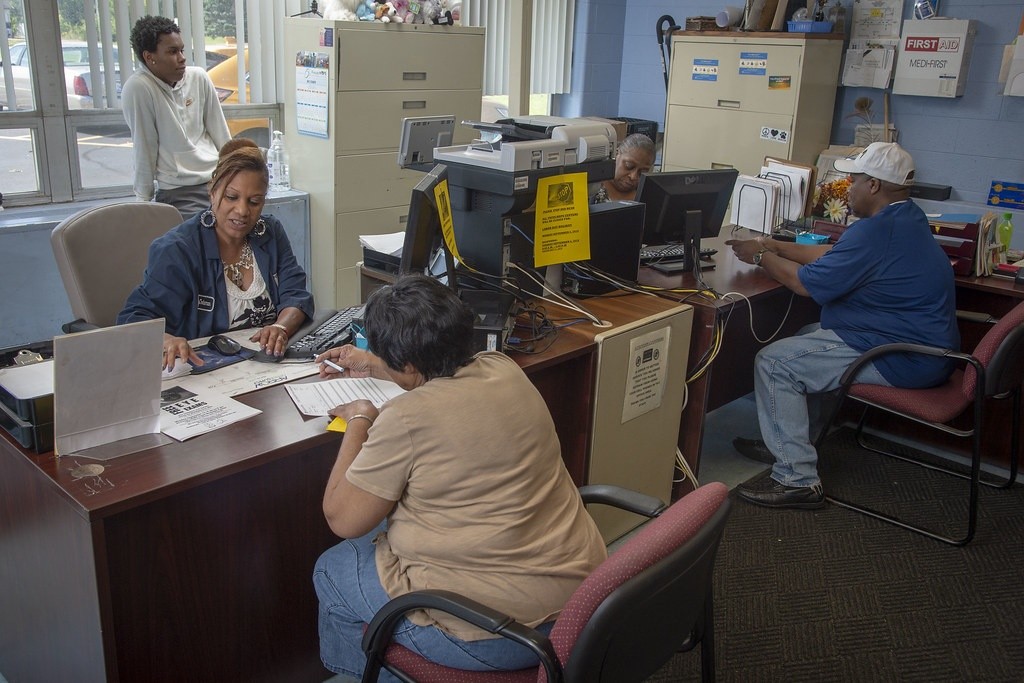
[732, 436, 777, 465]
[736, 477, 826, 509]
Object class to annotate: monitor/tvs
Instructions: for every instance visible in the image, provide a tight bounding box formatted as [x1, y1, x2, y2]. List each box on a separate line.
[635, 168, 740, 275]
[399, 162, 461, 299]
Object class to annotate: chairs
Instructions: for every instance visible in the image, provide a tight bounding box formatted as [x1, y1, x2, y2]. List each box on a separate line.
[50, 201, 184, 335]
[358, 481, 729, 682]
[813, 300, 1024, 548]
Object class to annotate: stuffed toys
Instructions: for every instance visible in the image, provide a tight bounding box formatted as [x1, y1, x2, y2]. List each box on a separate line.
[318, 0, 463, 26]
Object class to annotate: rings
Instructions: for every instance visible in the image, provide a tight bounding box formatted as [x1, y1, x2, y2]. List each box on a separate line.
[164, 349, 167, 353]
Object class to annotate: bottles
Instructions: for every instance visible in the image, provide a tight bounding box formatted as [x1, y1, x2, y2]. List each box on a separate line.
[267, 131, 290, 191]
[999, 212, 1013, 256]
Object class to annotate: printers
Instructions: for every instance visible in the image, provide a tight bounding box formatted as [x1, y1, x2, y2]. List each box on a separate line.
[395, 113, 618, 302]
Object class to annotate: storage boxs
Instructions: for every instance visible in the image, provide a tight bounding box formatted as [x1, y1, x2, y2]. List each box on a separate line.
[363, 246, 402, 274]
[608, 117, 657, 144]
[0, 340, 54, 425]
[0, 402, 54, 455]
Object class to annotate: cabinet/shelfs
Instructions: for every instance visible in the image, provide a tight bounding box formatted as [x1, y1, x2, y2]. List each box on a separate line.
[660, 30, 847, 229]
[929, 219, 981, 277]
[283, 16, 487, 311]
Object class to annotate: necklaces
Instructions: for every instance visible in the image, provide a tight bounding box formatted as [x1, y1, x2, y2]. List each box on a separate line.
[220, 238, 251, 287]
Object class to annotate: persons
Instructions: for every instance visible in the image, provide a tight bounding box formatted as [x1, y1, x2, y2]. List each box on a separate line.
[604, 133, 657, 202]
[314, 274, 610, 683]
[120, 13, 233, 223]
[113, 139, 317, 372]
[723, 141, 960, 511]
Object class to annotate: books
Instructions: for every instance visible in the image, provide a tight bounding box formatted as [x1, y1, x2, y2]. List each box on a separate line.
[977, 211, 1000, 276]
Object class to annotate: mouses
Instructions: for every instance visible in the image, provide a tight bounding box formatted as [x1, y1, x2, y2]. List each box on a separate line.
[208, 335, 241, 357]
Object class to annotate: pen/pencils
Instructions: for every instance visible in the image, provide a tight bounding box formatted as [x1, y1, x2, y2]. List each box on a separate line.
[312, 352, 344, 374]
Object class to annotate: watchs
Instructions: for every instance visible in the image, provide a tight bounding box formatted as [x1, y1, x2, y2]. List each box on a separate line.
[752, 248, 769, 266]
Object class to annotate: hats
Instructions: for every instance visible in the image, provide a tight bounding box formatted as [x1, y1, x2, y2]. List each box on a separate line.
[834, 141, 916, 186]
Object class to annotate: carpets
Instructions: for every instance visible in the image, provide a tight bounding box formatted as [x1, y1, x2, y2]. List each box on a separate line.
[643, 424, 1023, 683]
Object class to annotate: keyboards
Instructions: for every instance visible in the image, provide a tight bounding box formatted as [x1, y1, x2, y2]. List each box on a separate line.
[640, 245, 718, 266]
[285, 305, 367, 358]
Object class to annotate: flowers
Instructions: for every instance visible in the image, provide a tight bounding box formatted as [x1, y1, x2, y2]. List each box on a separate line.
[813, 176, 854, 224]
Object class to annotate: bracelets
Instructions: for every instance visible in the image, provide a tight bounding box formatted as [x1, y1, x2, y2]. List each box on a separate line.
[271, 324, 289, 335]
[346, 414, 374, 425]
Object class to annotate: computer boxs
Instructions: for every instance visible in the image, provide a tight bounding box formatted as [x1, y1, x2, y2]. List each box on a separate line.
[352, 288, 520, 360]
[561, 198, 645, 299]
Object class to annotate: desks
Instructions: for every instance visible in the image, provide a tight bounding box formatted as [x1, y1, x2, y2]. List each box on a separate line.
[0, 224, 1024, 683]
